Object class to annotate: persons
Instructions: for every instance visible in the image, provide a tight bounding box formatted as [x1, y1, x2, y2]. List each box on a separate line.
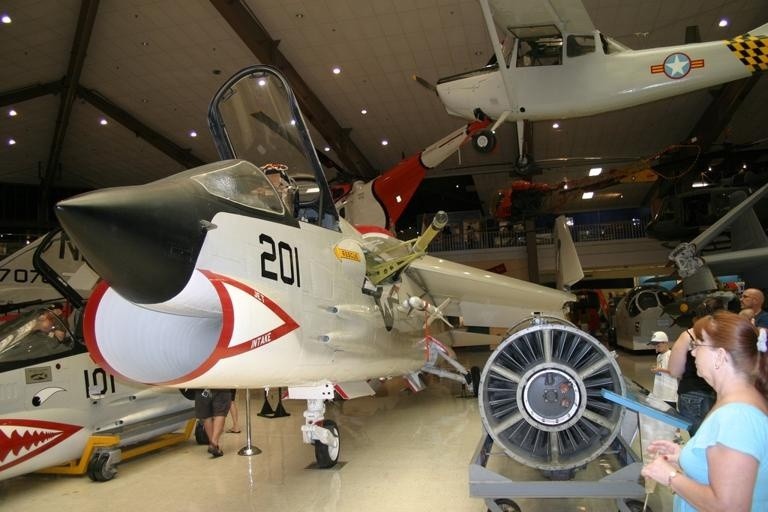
[47, 302, 68, 342]
[640, 288, 768, 512]
[467, 224, 474, 249]
[226, 389, 241, 433]
[195, 389, 231, 456]
[443, 224, 452, 239]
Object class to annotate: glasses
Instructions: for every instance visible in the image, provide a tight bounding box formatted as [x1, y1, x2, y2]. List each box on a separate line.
[688, 340, 728, 362]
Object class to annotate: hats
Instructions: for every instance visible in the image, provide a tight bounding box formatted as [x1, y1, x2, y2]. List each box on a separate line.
[647, 331, 669, 345]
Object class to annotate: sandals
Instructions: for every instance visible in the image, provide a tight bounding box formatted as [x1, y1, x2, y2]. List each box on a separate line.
[207, 442, 223, 456]
